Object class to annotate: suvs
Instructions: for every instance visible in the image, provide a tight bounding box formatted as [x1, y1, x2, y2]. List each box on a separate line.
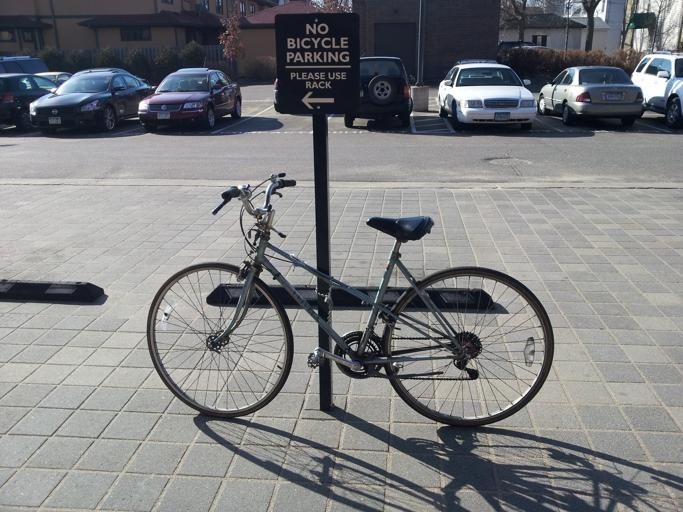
[629, 49, 683, 130]
[342, 55, 414, 129]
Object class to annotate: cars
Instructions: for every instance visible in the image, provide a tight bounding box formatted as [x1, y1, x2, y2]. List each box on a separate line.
[450, 40, 536, 84]
[0, 55, 244, 136]
[435, 62, 539, 132]
[537, 64, 647, 130]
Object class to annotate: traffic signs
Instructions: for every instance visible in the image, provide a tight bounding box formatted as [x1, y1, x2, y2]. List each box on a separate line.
[275, 13, 361, 118]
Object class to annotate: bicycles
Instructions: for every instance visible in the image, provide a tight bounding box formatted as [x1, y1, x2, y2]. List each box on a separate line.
[142, 171, 557, 430]
